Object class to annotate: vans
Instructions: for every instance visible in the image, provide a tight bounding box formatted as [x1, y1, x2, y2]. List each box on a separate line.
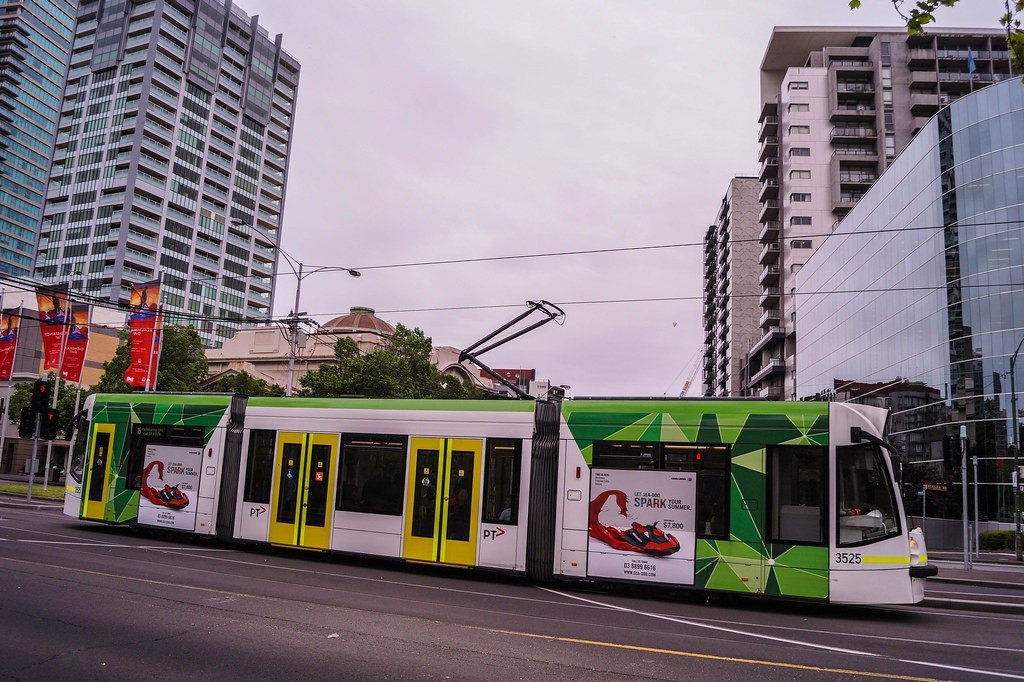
[1013, 512, 1024, 523]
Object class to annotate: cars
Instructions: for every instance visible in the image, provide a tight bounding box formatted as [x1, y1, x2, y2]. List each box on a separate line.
[61, 467, 82, 476]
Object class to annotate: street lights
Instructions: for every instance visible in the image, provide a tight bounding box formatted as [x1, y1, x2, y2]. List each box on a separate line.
[230, 218, 362, 396]
[538, 384, 571, 399]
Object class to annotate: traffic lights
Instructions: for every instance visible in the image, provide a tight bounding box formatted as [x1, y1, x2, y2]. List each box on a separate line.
[31, 381, 52, 411]
[42, 408, 59, 440]
[693, 448, 704, 468]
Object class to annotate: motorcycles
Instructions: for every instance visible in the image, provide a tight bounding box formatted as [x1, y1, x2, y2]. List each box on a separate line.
[160, 484, 190, 509]
[621, 521, 681, 556]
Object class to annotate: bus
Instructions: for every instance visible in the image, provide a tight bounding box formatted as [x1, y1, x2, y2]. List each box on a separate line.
[60, 395, 939, 609]
